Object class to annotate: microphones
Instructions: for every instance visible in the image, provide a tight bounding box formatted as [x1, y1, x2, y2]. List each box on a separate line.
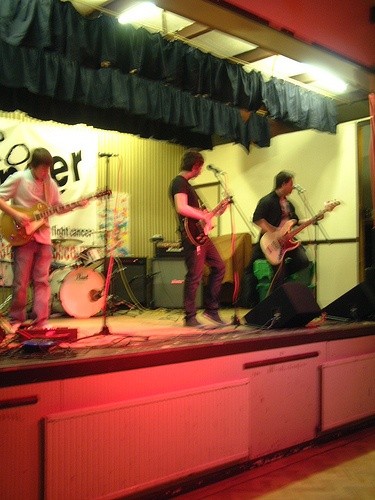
[293, 184, 305, 194]
[207, 165, 221, 173]
[99, 152, 119, 157]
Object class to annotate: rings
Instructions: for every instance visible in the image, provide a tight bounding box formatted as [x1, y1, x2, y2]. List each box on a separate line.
[20, 223, 23, 225]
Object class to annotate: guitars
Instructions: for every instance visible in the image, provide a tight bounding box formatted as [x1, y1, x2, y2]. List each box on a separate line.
[260, 199, 341, 265]
[183, 196, 234, 245]
[0, 189, 112, 246]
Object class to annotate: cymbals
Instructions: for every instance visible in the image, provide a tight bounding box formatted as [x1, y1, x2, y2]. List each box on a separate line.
[81, 244, 104, 247]
[52, 239, 83, 246]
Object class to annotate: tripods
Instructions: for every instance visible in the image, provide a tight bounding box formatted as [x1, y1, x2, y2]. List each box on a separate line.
[70, 156, 153, 341]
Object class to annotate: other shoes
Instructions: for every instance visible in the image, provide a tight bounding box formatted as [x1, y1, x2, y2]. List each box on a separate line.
[201, 311, 227, 326]
[186, 318, 206, 329]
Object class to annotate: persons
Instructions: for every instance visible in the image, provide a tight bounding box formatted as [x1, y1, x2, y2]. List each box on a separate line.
[168, 152, 227, 327]
[253, 170, 324, 286]
[0, 148, 89, 326]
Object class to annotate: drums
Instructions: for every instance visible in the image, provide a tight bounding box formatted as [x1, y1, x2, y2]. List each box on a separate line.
[49, 265, 106, 318]
[78, 247, 105, 268]
[0, 259, 14, 288]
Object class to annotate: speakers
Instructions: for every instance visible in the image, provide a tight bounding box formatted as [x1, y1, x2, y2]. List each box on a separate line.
[245, 283, 323, 328]
[152, 258, 204, 310]
[105, 256, 151, 308]
[323, 280, 375, 319]
